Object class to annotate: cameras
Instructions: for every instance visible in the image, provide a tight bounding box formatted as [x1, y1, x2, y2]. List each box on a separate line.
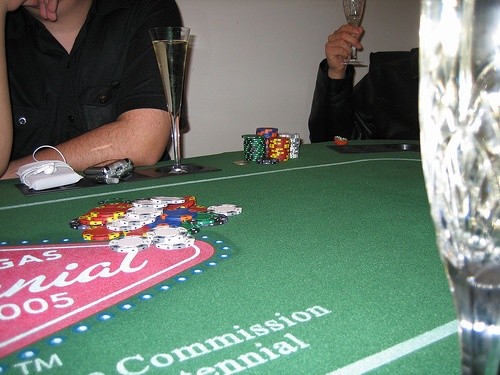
[84, 158, 135, 184]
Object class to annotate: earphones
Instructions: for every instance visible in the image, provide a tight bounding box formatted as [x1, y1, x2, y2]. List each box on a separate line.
[32, 163, 57, 175]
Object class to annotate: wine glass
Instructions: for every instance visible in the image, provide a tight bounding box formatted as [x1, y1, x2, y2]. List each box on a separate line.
[339, 0, 365, 66]
[150, 27, 202, 173]
[418, 0, 500, 375]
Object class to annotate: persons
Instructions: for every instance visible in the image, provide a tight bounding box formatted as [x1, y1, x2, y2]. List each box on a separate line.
[0, 0, 187, 180]
[308, 24, 419, 143]
